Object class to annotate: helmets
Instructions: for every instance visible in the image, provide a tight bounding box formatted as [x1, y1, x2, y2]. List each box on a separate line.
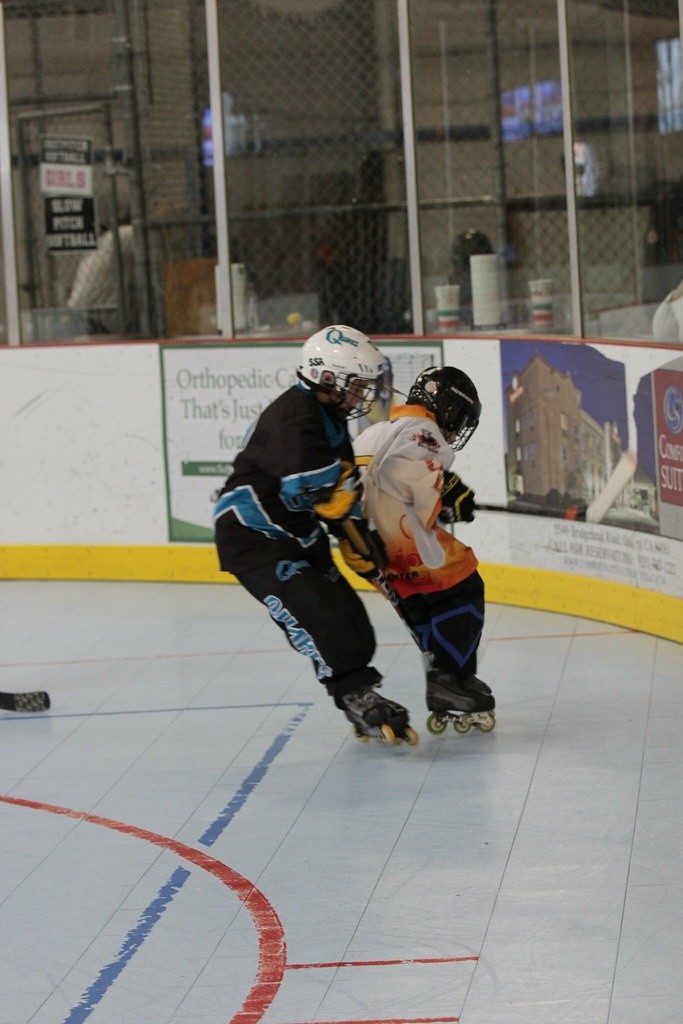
[296, 325, 389, 421]
[451, 230, 495, 273]
[407, 366, 481, 451]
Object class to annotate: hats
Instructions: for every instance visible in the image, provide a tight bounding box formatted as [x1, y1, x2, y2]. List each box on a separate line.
[91, 171, 133, 227]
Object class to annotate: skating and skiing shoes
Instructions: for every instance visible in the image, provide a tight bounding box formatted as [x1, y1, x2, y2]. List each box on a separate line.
[426, 668, 497, 734]
[336, 689, 418, 748]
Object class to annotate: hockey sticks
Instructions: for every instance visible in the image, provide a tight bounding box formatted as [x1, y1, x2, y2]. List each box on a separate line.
[341, 520, 422, 649]
[0, 690, 52, 713]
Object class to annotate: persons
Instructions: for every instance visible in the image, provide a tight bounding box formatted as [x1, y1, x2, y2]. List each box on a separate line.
[65, 158, 188, 339]
[214, 324, 418, 748]
[327, 365, 497, 733]
[652, 282, 683, 345]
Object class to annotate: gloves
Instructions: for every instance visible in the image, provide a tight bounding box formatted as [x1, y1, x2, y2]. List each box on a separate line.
[338, 528, 382, 580]
[438, 471, 474, 525]
[312, 462, 365, 525]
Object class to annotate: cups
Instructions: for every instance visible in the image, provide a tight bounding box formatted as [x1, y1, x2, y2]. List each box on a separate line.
[529, 279, 556, 334]
[434, 285, 461, 335]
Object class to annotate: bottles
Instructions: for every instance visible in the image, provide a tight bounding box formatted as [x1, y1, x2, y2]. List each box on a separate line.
[286, 311, 304, 332]
